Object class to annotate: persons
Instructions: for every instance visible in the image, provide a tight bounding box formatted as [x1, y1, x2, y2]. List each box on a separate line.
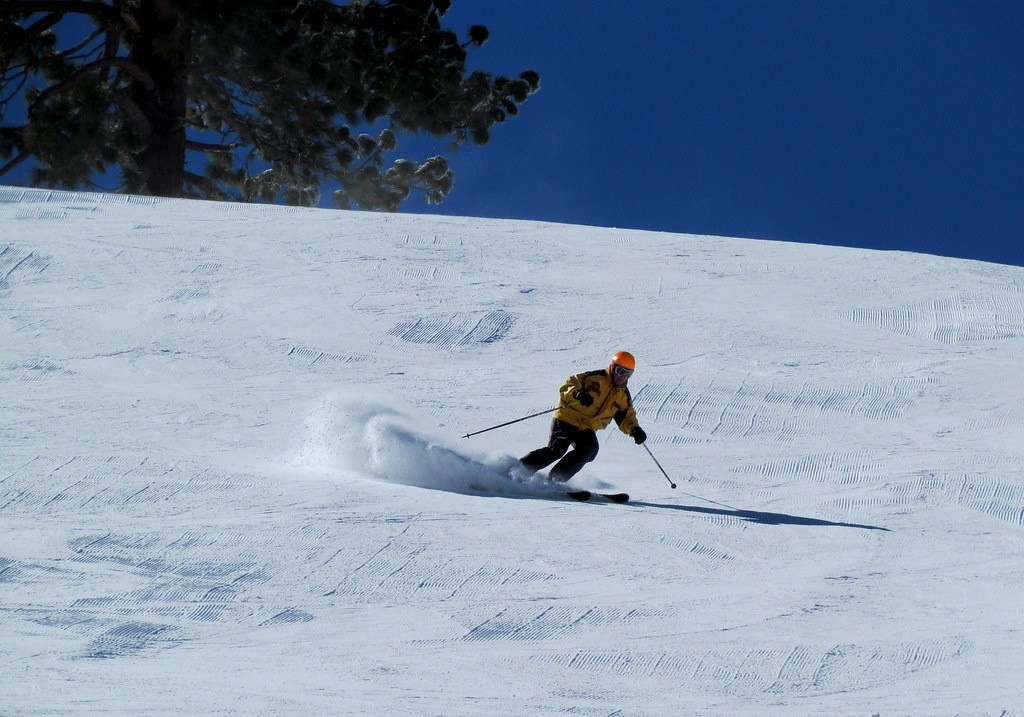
[510, 351, 646, 484]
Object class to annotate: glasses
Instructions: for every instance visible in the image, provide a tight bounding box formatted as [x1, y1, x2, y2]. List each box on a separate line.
[610, 361, 634, 380]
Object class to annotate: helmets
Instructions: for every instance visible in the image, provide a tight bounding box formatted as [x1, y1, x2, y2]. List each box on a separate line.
[613, 351, 635, 369]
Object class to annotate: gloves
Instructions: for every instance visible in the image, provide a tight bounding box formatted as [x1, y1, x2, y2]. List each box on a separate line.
[572, 390, 594, 408]
[630, 425, 647, 445]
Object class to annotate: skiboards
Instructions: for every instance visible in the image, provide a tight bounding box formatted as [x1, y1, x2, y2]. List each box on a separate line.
[568, 490, 629, 505]
